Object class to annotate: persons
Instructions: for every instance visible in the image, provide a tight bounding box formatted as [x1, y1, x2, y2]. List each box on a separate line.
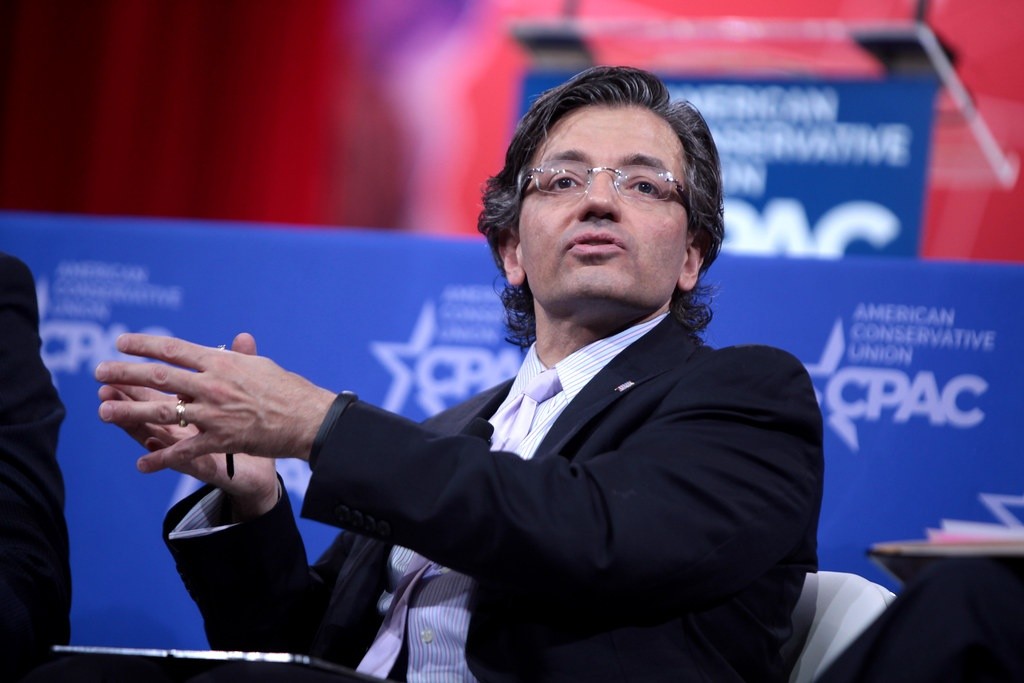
[0, 251, 73, 683]
[96, 65, 825, 683]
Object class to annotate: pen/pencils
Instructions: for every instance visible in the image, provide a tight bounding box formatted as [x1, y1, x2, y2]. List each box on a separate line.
[226, 452, 234, 480]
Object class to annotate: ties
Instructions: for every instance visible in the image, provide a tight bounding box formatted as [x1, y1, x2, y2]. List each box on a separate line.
[357, 369, 563, 681]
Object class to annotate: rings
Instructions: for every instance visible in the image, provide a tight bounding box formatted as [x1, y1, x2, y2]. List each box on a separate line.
[176, 399, 187, 429]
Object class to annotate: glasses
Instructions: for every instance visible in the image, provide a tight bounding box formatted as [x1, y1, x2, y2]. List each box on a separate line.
[521, 164, 689, 213]
